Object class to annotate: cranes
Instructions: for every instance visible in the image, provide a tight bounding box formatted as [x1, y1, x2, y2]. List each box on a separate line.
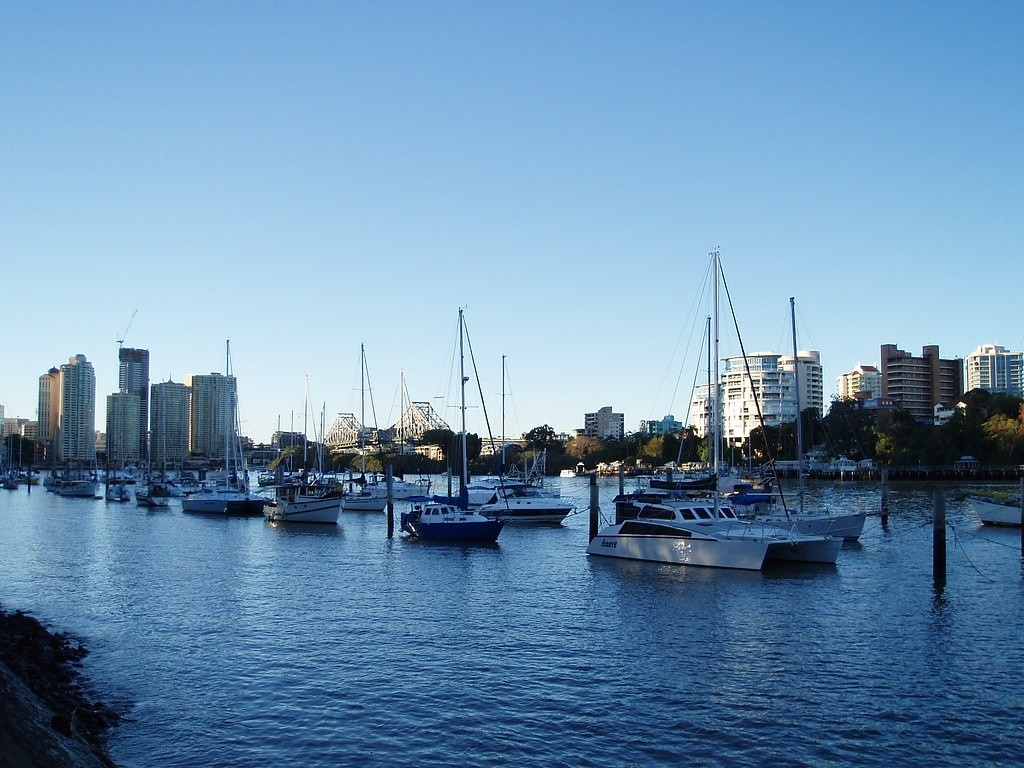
[116, 309, 138, 347]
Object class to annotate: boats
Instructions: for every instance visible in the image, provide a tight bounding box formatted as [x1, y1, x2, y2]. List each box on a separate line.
[965, 495, 1021, 529]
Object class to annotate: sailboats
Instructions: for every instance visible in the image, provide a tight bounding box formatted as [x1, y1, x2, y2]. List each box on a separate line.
[0, 303, 579, 543]
[585, 245, 868, 573]
